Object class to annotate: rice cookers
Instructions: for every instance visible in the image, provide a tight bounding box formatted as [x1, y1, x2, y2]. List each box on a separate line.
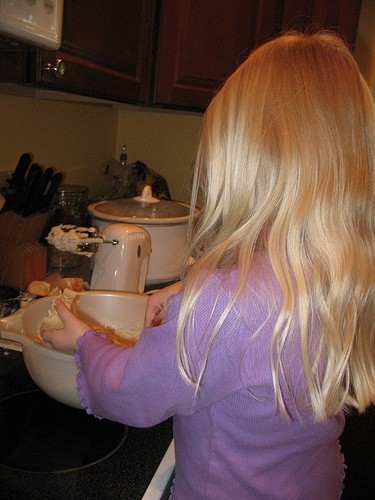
[87, 185, 202, 287]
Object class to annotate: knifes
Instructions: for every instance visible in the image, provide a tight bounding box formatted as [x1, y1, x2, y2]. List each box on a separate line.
[1, 152, 64, 218]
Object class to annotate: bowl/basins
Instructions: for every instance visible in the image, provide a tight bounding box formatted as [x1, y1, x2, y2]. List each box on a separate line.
[0, 290, 162, 409]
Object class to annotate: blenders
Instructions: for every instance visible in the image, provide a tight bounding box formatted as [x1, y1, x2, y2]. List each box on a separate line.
[47, 223, 151, 294]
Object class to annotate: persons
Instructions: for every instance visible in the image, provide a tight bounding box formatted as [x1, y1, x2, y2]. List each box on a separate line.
[42, 30, 375, 500]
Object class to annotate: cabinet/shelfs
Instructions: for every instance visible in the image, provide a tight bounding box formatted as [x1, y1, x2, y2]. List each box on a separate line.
[0, 0, 363, 113]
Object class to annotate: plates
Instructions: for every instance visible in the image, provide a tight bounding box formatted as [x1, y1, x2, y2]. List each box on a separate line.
[0, 311, 23, 353]
